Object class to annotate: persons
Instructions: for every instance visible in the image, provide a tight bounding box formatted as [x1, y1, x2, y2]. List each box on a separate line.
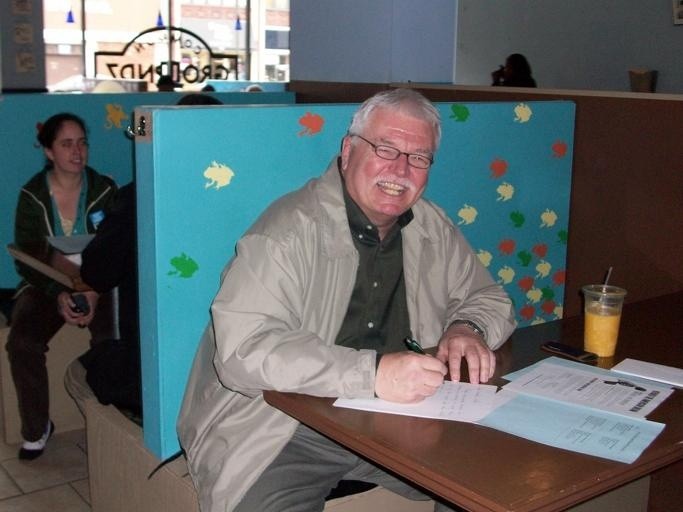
[63, 95, 224, 452]
[175, 88, 519, 512]
[491, 53, 537, 88]
[5, 112, 119, 461]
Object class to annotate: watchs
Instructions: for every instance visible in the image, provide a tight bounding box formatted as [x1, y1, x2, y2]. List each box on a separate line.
[448, 320, 484, 338]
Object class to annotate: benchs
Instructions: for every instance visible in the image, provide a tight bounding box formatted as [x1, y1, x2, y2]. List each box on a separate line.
[82, 99, 575, 512]
[0, 91, 296, 452]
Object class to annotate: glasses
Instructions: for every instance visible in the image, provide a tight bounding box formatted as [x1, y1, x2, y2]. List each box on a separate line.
[348, 133, 434, 170]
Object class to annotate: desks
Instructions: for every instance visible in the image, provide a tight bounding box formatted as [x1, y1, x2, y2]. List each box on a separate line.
[266, 288, 682, 512]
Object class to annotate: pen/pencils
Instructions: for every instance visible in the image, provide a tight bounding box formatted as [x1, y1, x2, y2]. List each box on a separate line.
[404, 335, 447, 385]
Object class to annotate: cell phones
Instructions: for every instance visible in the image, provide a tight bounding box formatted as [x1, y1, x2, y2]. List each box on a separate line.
[541, 339, 598, 362]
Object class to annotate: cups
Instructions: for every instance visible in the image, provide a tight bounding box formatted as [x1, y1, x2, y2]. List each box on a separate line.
[578, 284, 627, 359]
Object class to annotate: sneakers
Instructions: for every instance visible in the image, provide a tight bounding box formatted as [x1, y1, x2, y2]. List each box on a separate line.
[17, 417, 55, 462]
